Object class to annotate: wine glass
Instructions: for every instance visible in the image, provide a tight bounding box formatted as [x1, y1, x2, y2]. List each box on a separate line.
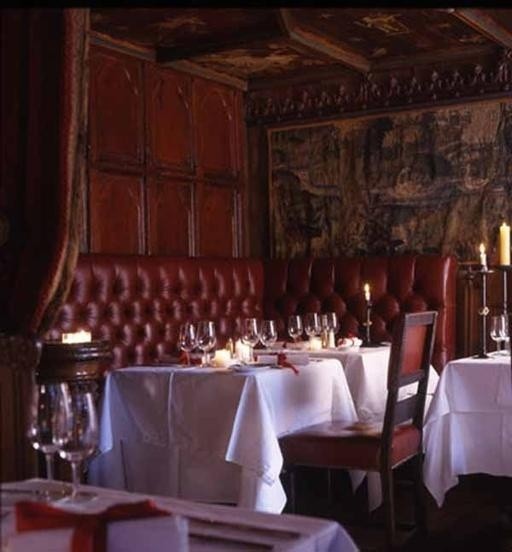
[240, 317, 259, 367]
[23, 381, 72, 501]
[259, 319, 278, 353]
[195, 319, 218, 370]
[51, 391, 101, 508]
[489, 314, 507, 359]
[286, 314, 304, 344]
[177, 322, 198, 369]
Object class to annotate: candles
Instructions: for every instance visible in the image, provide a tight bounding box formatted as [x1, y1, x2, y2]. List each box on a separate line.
[479, 221, 509, 270]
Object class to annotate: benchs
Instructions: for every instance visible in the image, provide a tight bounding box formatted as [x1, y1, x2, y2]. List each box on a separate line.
[40, 256, 457, 375]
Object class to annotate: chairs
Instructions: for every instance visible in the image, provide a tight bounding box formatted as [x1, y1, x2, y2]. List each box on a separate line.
[283, 308, 437, 552]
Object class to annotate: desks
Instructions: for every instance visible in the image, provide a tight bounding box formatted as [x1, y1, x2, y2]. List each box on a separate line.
[104, 339, 438, 513]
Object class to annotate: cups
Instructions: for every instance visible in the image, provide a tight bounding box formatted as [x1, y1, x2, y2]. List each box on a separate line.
[316, 314, 329, 335]
[323, 311, 341, 336]
[304, 311, 323, 338]
